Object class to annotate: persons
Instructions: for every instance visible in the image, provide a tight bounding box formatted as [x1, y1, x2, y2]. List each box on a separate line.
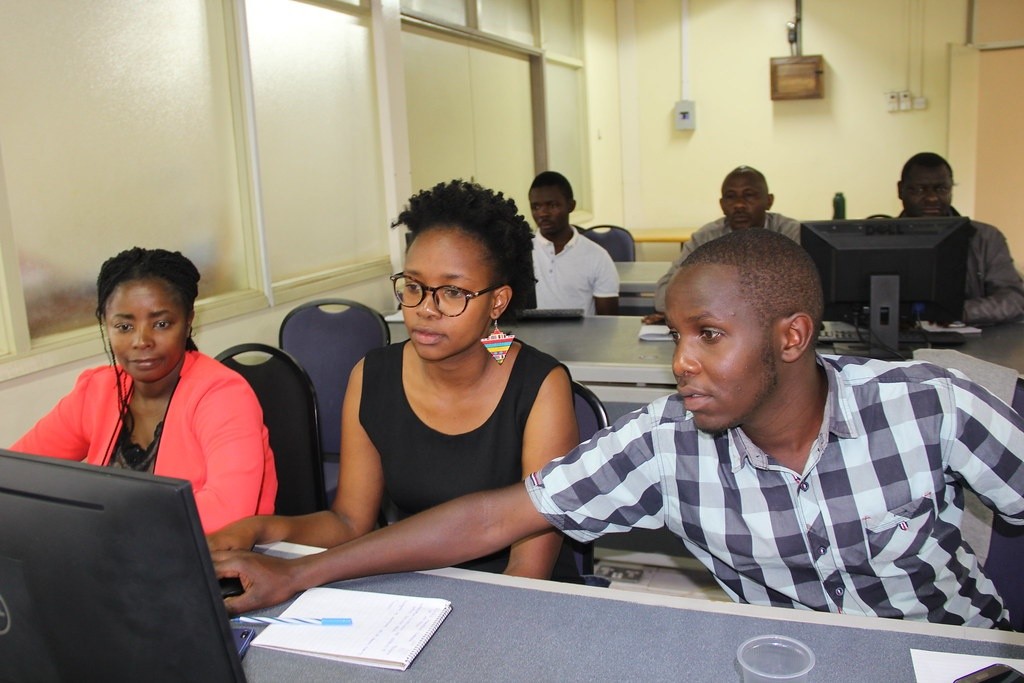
[203, 180, 577, 581]
[527, 171, 620, 316]
[1, 245, 279, 536]
[186, 228, 1024, 632]
[641, 165, 801, 325]
[898, 152, 1023, 325]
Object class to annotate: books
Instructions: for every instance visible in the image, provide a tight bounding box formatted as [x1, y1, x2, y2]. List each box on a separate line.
[249, 587, 453, 672]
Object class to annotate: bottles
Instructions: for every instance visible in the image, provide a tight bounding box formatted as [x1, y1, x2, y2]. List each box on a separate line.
[833, 192, 845, 218]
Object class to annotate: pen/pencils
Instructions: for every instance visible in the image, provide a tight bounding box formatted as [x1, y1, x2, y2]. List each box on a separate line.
[231, 616, 352, 625]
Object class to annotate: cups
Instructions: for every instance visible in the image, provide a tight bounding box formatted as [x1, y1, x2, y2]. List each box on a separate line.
[737, 632, 815, 683]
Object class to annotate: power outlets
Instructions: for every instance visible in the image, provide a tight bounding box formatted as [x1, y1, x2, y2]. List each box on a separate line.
[913, 97, 927, 111]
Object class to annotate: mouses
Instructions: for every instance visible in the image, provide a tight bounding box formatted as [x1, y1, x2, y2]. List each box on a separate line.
[215, 576, 245, 600]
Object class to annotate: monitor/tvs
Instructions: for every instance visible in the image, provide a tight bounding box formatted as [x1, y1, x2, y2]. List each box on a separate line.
[405, 227, 538, 326]
[800, 217, 969, 361]
[0, 449, 247, 683]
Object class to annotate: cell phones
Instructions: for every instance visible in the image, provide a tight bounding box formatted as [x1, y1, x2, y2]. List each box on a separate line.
[231, 627, 255, 660]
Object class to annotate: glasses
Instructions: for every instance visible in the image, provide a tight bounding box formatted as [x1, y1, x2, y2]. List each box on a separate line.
[390, 272, 512, 317]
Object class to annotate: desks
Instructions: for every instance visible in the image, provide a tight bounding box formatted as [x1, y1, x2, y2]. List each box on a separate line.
[211, 263, 1024, 683]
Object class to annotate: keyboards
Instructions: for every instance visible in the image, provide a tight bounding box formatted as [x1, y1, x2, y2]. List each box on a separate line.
[816, 330, 967, 345]
[519, 309, 586, 322]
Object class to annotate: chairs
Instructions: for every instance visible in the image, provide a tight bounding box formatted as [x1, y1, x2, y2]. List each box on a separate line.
[278, 298, 391, 529]
[568, 379, 613, 589]
[912, 347, 1024, 637]
[213, 343, 327, 517]
[581, 225, 637, 263]
